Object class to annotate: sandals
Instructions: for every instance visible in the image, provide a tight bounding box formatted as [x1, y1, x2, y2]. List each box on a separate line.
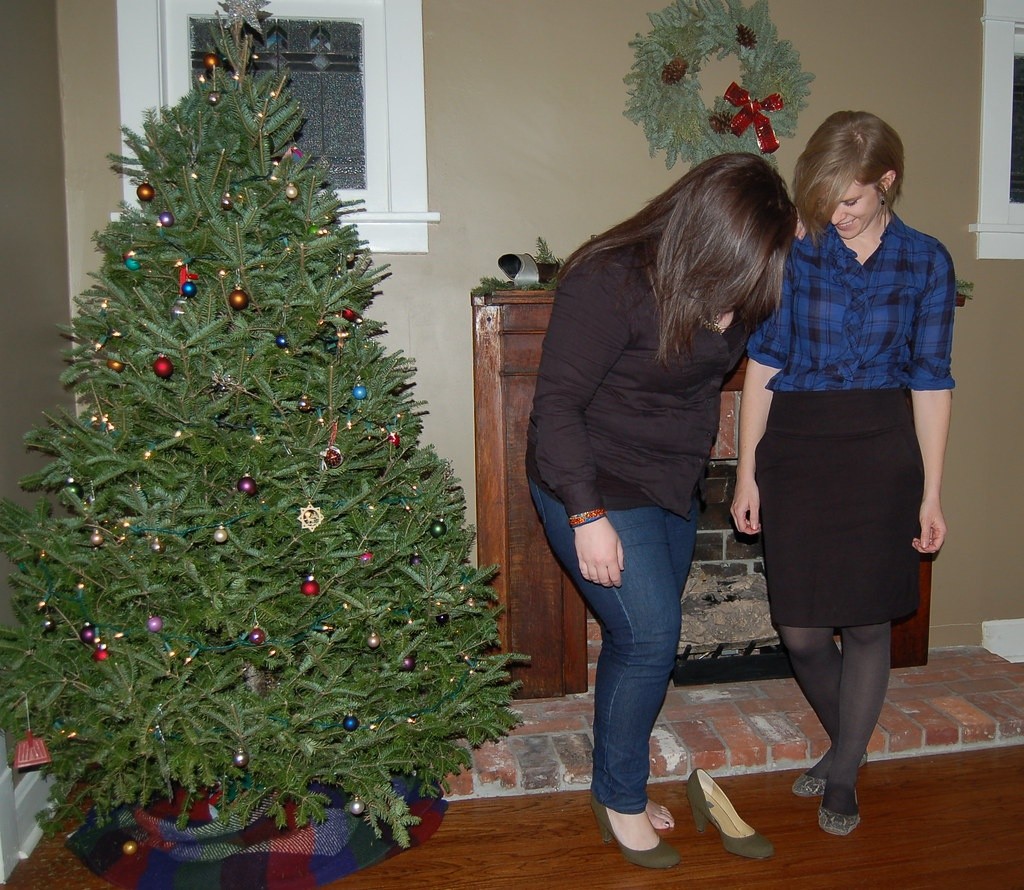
[792, 747, 867, 798]
[818, 796, 860, 836]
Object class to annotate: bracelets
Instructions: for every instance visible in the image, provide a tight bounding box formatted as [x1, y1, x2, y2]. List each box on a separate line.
[569, 508, 607, 527]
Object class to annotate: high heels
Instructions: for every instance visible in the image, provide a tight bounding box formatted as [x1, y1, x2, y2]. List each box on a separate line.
[687, 768, 774, 858]
[590, 791, 681, 868]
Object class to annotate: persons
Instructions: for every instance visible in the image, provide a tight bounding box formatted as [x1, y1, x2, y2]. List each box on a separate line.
[730, 110, 957, 835]
[525, 152, 808, 869]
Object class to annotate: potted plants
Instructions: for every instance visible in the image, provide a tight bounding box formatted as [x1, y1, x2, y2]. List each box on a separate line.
[534, 236, 560, 283]
